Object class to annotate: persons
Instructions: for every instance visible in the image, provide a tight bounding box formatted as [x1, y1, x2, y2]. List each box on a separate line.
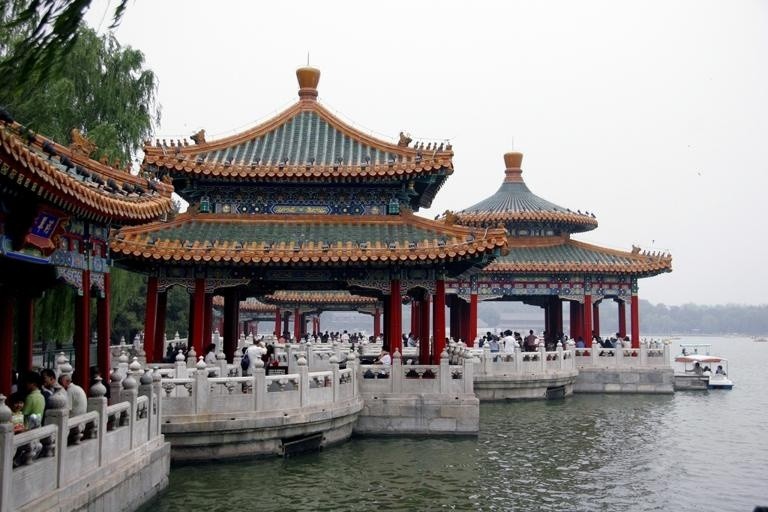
[445, 330, 632, 360]
[5, 369, 87, 467]
[402, 333, 419, 347]
[241, 335, 278, 376]
[203, 343, 219, 377]
[166, 342, 187, 363]
[90, 366, 111, 407]
[682, 348, 727, 375]
[271, 329, 391, 378]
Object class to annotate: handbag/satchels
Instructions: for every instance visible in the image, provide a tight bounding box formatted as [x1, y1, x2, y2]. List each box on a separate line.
[241, 355, 250, 370]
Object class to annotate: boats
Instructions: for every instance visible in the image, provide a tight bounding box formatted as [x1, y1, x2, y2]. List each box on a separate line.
[680, 344, 712, 356]
[674, 354, 734, 391]
